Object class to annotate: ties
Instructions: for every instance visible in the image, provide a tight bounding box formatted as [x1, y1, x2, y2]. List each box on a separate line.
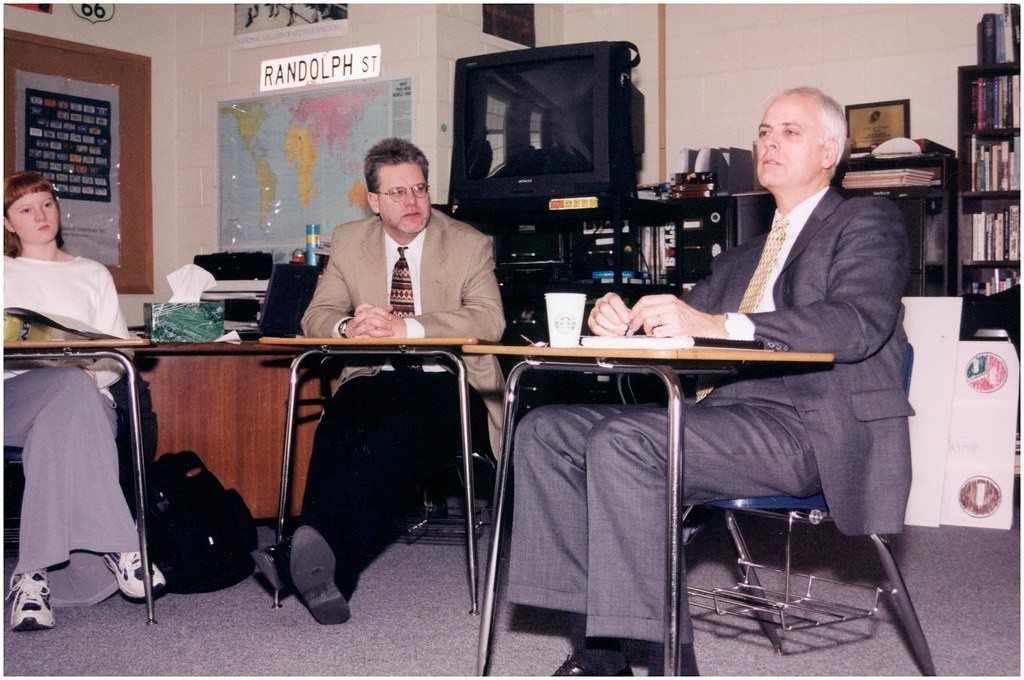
[695, 216, 790, 403]
[390, 247, 422, 371]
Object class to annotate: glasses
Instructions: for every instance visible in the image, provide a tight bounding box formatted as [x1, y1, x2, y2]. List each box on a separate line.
[375, 183, 430, 202]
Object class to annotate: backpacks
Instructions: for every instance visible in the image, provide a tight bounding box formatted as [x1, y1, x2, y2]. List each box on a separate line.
[148, 451, 258, 595]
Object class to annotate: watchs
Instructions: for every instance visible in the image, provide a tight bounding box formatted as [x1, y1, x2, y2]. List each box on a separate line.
[338, 318, 353, 338]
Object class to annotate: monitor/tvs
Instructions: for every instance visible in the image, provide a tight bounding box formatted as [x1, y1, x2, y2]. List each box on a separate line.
[452, 41, 645, 200]
[258, 264, 320, 333]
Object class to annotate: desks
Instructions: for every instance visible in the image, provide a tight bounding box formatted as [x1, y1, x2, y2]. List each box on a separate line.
[461, 345, 834, 676]
[4, 339, 158, 625]
[259, 335, 503, 617]
[133, 341, 338, 519]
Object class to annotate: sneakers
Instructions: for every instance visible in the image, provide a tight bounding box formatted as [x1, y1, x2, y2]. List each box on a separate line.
[5, 568, 55, 631]
[103, 551, 166, 599]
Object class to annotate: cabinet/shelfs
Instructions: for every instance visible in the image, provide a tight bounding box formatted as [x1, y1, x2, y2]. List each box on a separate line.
[735, 154, 958, 296]
[953, 60, 1021, 296]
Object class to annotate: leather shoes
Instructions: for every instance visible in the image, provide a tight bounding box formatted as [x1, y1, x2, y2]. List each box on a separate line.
[552, 653, 633, 676]
[250, 526, 351, 626]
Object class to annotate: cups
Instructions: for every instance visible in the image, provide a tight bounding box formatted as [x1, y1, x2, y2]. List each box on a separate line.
[306, 224, 320, 266]
[544, 293, 587, 349]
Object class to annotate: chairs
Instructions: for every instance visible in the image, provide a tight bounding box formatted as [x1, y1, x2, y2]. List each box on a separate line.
[683, 343, 937, 676]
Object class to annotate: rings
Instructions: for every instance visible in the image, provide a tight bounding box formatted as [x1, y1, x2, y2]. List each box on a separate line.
[657, 315, 662, 326]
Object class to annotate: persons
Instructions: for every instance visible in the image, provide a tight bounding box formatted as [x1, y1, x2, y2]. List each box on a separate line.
[507, 87, 916, 677]
[251, 135, 511, 625]
[4, 176, 167, 631]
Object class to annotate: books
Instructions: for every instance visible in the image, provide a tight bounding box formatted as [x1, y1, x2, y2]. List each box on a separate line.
[962, 4, 1021, 296]
[578, 336, 694, 350]
[842, 169, 935, 188]
[637, 222, 676, 285]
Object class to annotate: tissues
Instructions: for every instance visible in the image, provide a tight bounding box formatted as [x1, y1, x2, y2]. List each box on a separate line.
[143, 264, 226, 341]
